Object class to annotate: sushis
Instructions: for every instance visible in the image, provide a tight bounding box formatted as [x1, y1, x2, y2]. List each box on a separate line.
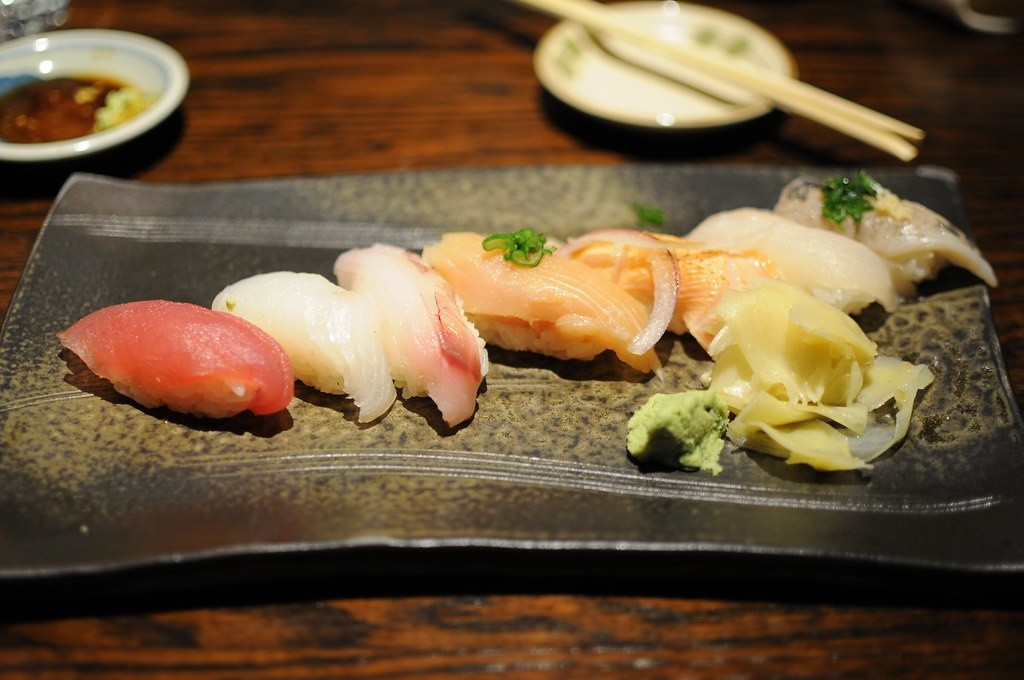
[56, 169, 998, 429]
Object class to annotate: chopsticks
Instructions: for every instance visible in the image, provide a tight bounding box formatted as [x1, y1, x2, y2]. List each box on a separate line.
[511, 0, 925, 164]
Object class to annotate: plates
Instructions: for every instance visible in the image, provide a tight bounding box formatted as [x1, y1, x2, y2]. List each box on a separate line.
[1, 167, 1024, 575]
[0, 28, 190, 161]
[535, 2, 798, 128]
